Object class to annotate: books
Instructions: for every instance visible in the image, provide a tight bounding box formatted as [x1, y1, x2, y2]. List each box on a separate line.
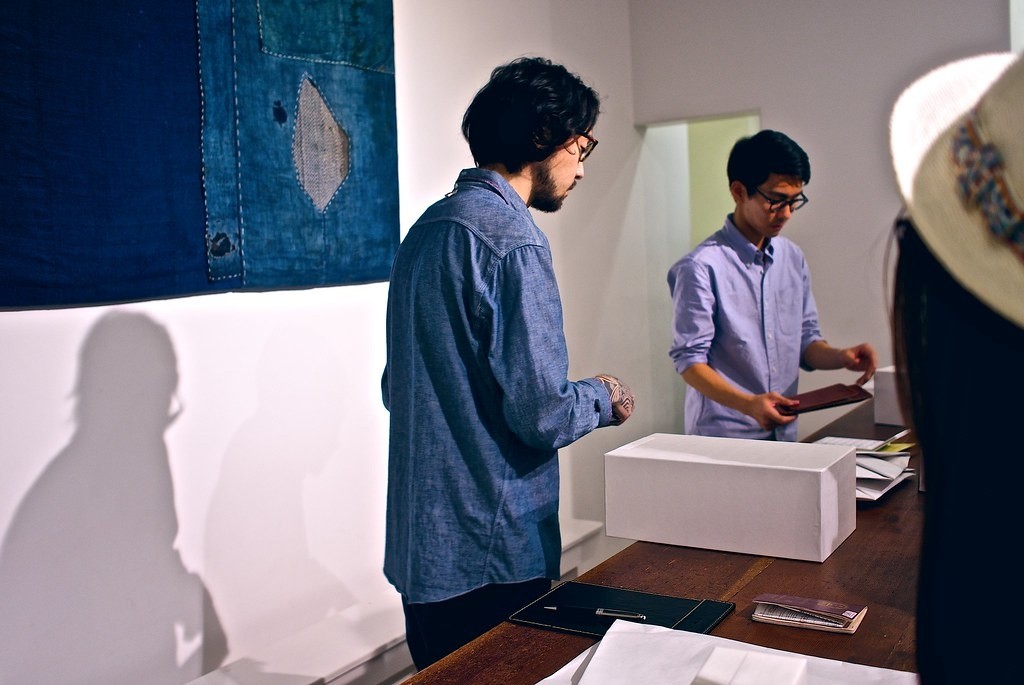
[813, 427, 918, 503]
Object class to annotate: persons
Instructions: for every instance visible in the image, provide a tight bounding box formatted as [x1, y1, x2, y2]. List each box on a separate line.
[379, 56, 635, 669]
[665, 131, 875, 444]
[881, 48, 1024, 685]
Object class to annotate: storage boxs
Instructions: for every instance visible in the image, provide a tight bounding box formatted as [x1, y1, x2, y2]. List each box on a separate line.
[603, 432, 857, 563]
[873, 366, 904, 426]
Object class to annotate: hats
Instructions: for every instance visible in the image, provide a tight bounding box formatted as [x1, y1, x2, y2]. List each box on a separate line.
[889, 53, 1024, 326]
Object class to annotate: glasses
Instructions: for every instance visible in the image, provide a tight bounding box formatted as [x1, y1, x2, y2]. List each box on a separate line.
[577, 130, 598, 162]
[755, 190, 808, 212]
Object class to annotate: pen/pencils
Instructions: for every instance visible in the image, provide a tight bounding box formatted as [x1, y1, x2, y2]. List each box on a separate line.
[543, 604, 645, 622]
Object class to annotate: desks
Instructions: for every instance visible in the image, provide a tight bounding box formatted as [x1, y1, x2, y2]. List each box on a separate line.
[390, 397, 920, 685]
